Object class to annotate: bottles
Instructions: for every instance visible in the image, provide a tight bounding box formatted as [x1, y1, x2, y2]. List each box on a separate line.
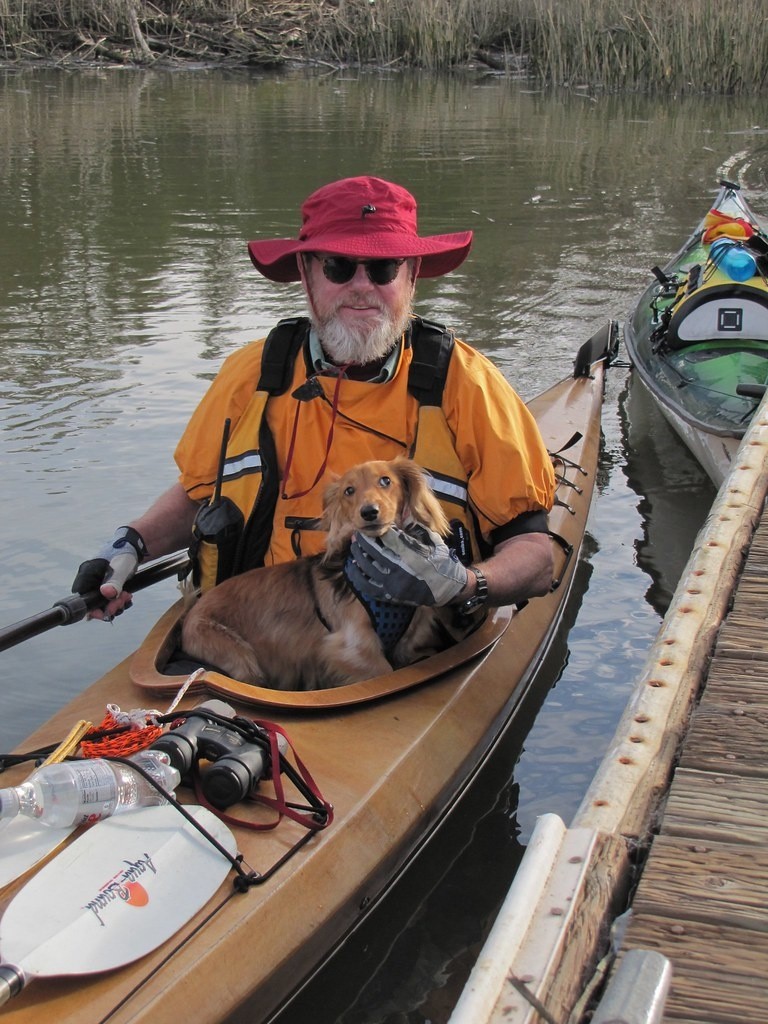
[0, 749, 181, 829]
[710, 238, 756, 282]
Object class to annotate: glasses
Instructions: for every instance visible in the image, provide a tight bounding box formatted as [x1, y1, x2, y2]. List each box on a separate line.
[310, 249, 407, 286]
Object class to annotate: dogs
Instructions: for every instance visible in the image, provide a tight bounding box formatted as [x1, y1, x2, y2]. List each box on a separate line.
[178, 454, 455, 691]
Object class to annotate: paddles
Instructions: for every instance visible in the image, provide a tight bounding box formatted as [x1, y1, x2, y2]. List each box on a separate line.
[0, 547, 192, 653]
[0, 802, 238, 1008]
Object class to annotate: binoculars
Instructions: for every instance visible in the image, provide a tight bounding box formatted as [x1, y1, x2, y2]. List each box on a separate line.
[147, 700, 287, 812]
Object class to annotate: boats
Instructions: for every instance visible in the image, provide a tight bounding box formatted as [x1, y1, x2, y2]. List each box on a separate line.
[1, 321, 610, 1023]
[623, 365, 716, 613]
[621, 179, 767, 493]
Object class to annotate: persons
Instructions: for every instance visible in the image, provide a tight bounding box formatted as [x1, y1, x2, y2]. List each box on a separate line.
[71, 175, 574, 671]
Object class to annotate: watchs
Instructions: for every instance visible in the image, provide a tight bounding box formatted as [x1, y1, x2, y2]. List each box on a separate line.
[456, 567, 487, 616]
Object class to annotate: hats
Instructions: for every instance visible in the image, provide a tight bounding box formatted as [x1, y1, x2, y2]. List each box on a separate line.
[248, 178, 474, 284]
